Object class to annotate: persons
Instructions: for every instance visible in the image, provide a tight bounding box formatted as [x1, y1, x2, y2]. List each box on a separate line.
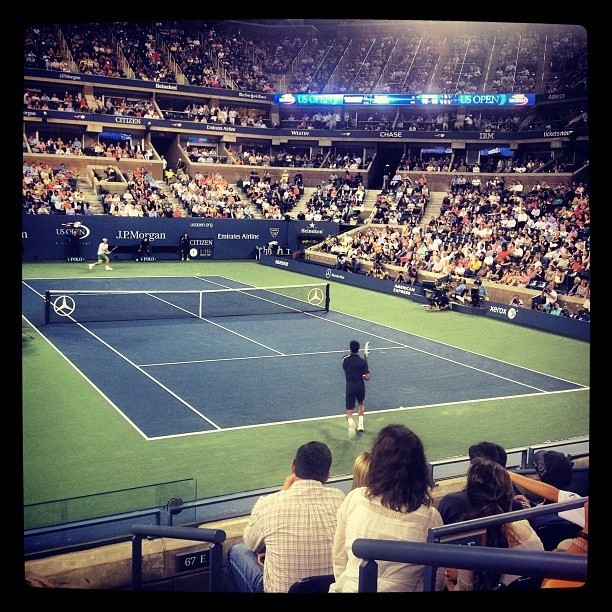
[101, 21, 588, 93]
[181, 233, 192, 261]
[182, 145, 232, 164]
[524, 448, 573, 509]
[438, 442, 534, 526]
[444, 460, 546, 591]
[234, 168, 304, 219]
[543, 501, 588, 589]
[286, 111, 585, 131]
[401, 153, 450, 172]
[452, 158, 567, 172]
[160, 155, 256, 219]
[27, 137, 155, 160]
[371, 173, 432, 226]
[227, 441, 346, 594]
[328, 424, 446, 593]
[97, 165, 175, 217]
[88, 238, 114, 271]
[136, 238, 154, 262]
[22, 88, 160, 120]
[351, 449, 373, 491]
[341, 339, 370, 439]
[68, 230, 84, 262]
[236, 148, 324, 168]
[429, 175, 590, 226]
[319, 226, 589, 331]
[179, 101, 269, 127]
[325, 152, 373, 169]
[21, 162, 94, 216]
[297, 169, 367, 225]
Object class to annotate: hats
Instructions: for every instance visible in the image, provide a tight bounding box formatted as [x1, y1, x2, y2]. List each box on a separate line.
[532, 450, 573, 489]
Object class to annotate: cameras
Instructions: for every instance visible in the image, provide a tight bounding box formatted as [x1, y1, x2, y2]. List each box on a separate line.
[543, 288, 548, 293]
[514, 298, 520, 304]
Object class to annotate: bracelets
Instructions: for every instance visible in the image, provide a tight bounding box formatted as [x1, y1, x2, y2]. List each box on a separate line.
[571, 538, 588, 552]
[577, 531, 588, 541]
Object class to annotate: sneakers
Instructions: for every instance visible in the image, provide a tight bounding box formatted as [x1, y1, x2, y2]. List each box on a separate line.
[105, 267, 113, 271]
[89, 264, 92, 269]
[347, 421, 356, 440]
[358, 423, 365, 432]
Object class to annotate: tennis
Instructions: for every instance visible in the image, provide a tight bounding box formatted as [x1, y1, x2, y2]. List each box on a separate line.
[196, 273, 199, 276]
